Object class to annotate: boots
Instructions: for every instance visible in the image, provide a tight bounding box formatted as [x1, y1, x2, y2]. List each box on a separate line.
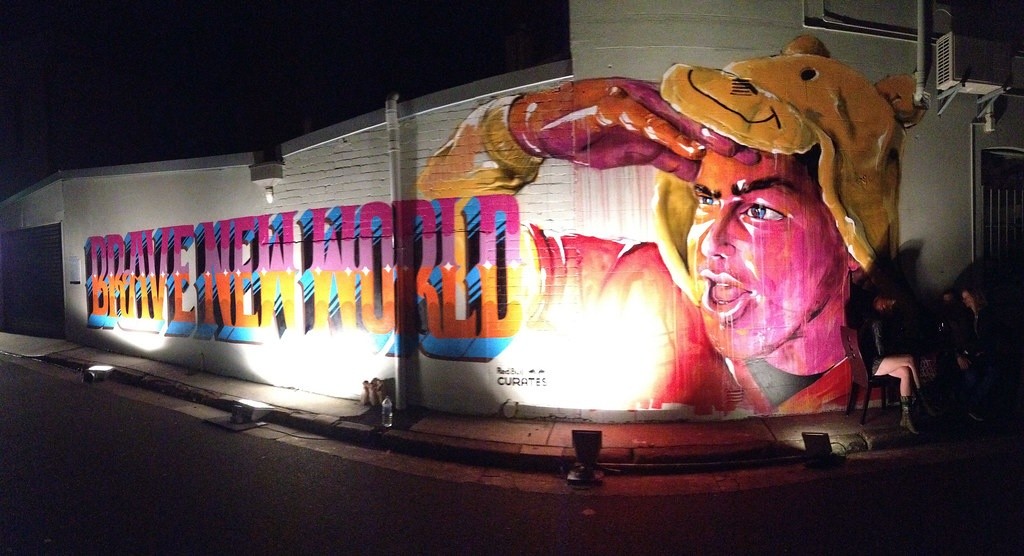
[913, 386, 940, 417]
[900, 396, 920, 435]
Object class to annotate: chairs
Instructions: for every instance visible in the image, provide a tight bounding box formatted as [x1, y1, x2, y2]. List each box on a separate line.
[838, 325, 900, 426]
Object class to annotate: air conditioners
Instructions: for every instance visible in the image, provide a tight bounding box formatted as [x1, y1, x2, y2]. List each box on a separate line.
[936, 30, 1014, 95]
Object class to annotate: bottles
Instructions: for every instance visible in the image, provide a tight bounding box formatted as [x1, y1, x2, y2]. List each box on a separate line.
[381, 395, 393, 427]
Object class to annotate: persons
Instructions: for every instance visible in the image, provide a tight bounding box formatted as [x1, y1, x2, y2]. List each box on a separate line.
[859, 294, 942, 434]
[953, 286, 999, 422]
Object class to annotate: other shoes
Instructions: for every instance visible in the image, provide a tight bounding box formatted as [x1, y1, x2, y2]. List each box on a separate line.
[967, 408, 984, 421]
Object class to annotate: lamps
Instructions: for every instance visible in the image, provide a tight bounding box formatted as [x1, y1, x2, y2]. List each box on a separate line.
[232, 398, 276, 422]
[802, 429, 833, 456]
[572, 429, 603, 464]
[83, 363, 115, 381]
